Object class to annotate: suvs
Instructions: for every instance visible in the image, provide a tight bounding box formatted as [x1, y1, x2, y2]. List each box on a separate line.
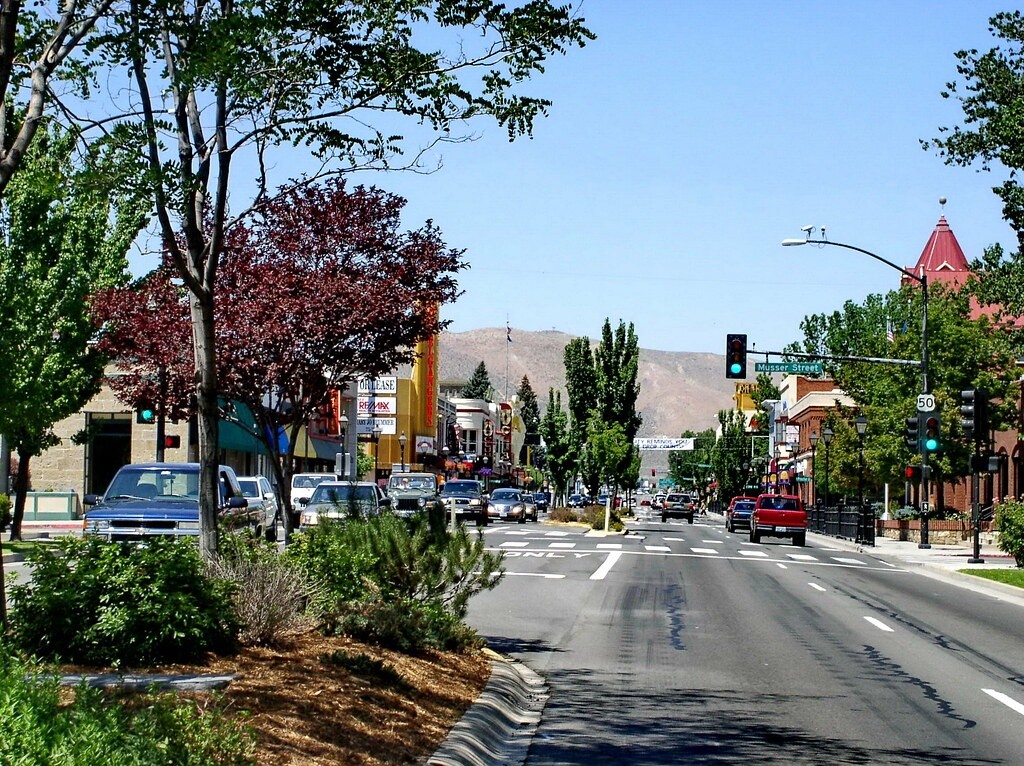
[660, 492, 695, 524]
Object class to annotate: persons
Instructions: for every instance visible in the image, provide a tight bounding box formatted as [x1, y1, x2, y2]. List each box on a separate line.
[420, 478, 434, 489]
[701, 500, 707, 516]
[398, 478, 411, 488]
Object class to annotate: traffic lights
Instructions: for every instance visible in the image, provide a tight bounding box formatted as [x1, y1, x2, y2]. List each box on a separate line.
[905, 465, 922, 481]
[651, 469, 655, 477]
[983, 455, 1001, 474]
[920, 413, 940, 453]
[136, 397, 155, 424]
[676, 458, 682, 472]
[726, 334, 747, 378]
[166, 436, 181, 449]
[961, 389, 981, 437]
[905, 413, 921, 452]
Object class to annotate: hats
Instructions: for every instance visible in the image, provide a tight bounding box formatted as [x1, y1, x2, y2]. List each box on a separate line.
[403, 478, 408, 482]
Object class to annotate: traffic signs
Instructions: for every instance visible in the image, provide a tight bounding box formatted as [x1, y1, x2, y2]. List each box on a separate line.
[755, 363, 823, 372]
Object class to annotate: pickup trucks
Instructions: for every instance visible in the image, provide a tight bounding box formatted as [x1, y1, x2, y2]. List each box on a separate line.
[748, 493, 807, 546]
[83, 463, 261, 555]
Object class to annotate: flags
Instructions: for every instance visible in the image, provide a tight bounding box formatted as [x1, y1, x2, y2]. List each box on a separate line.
[508, 328, 512, 343]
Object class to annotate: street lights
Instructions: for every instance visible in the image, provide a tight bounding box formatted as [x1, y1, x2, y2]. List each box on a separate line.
[773, 446, 782, 495]
[790, 438, 800, 495]
[782, 238, 929, 549]
[750, 455, 770, 494]
[372, 424, 383, 483]
[808, 430, 819, 505]
[853, 411, 868, 543]
[743, 460, 749, 496]
[821, 425, 835, 508]
[421, 442, 550, 491]
[398, 430, 407, 472]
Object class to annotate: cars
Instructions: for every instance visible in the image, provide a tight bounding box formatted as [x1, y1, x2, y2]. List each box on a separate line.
[220, 473, 549, 540]
[691, 499, 699, 513]
[566, 489, 666, 508]
[724, 496, 757, 532]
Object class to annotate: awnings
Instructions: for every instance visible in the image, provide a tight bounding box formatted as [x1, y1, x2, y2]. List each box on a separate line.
[216, 393, 345, 462]
